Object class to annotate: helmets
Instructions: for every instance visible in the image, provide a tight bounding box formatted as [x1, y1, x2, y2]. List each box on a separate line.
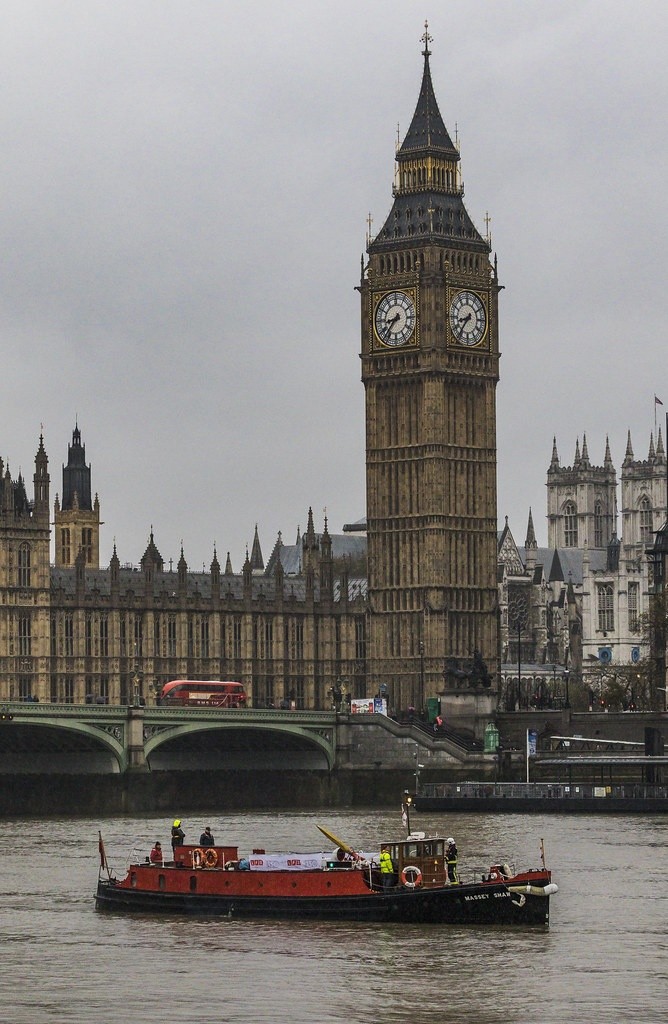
[447, 837, 456, 845]
[173, 820, 181, 827]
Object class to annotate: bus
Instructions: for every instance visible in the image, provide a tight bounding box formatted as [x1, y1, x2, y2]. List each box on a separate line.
[158, 679, 249, 708]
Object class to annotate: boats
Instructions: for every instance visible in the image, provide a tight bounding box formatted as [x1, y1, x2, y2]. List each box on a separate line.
[88, 784, 558, 935]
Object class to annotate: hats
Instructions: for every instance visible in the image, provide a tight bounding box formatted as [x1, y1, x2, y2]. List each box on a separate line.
[156, 841, 161, 846]
[206, 827, 211, 831]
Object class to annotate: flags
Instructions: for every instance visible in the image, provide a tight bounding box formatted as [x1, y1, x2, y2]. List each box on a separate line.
[401, 803, 407, 827]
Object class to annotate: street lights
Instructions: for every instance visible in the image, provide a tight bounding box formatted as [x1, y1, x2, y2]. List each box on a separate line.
[129, 663, 143, 708]
[330, 678, 346, 770]
[564, 667, 572, 710]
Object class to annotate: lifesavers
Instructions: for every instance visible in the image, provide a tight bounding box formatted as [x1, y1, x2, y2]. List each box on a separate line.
[190, 848, 204, 867]
[204, 849, 218, 868]
[400, 866, 423, 888]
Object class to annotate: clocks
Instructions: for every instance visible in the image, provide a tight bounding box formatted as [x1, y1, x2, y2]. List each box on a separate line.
[375, 291, 415, 347]
[450, 291, 487, 347]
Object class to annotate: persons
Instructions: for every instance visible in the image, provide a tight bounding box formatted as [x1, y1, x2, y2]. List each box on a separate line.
[27, 694, 39, 702]
[444, 838, 458, 885]
[150, 841, 162, 866]
[171, 820, 185, 868]
[200, 827, 214, 845]
[380, 845, 394, 895]
[432, 718, 438, 732]
